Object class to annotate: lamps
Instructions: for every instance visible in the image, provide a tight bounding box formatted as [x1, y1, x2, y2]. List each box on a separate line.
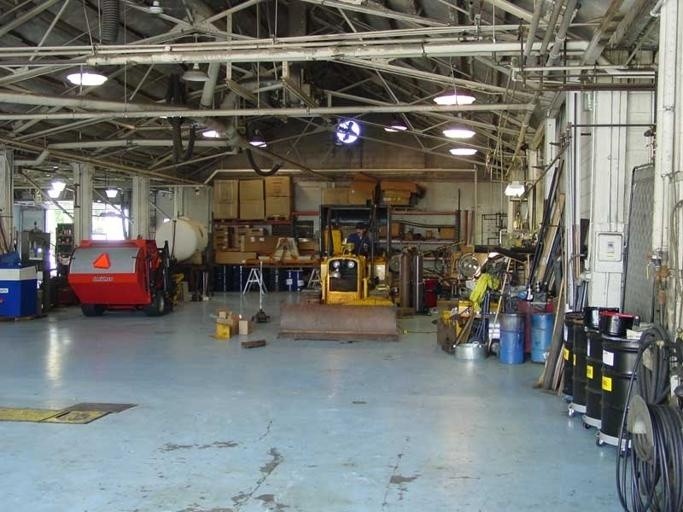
[383, 119, 406, 133]
[66, 67, 108, 86]
[248, 135, 267, 148]
[432, 89, 476, 138]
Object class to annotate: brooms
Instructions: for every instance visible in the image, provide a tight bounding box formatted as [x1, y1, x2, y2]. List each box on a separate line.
[533, 276, 565, 388]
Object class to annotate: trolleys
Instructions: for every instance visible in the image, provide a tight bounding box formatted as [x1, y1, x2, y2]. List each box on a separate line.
[555, 305, 653, 460]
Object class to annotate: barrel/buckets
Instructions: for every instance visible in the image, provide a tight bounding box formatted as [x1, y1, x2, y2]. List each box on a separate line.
[530, 312, 557, 364]
[563, 311, 640, 450]
[424, 278, 438, 307]
[498, 313, 526, 363]
[284, 269, 303, 291]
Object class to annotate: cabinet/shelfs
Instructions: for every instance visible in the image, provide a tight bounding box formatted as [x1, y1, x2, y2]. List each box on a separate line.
[318, 205, 460, 298]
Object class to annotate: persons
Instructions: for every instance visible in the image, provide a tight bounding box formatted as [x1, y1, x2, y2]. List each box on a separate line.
[348, 222, 372, 257]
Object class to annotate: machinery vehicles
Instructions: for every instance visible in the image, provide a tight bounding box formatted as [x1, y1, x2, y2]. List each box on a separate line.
[272, 197, 400, 343]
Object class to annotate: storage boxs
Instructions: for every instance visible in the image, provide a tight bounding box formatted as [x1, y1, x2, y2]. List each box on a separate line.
[322, 173, 417, 206]
[212, 223, 264, 252]
[215, 307, 239, 338]
[212, 175, 293, 220]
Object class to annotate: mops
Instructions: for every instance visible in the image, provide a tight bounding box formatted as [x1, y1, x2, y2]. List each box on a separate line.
[250, 260, 271, 323]
